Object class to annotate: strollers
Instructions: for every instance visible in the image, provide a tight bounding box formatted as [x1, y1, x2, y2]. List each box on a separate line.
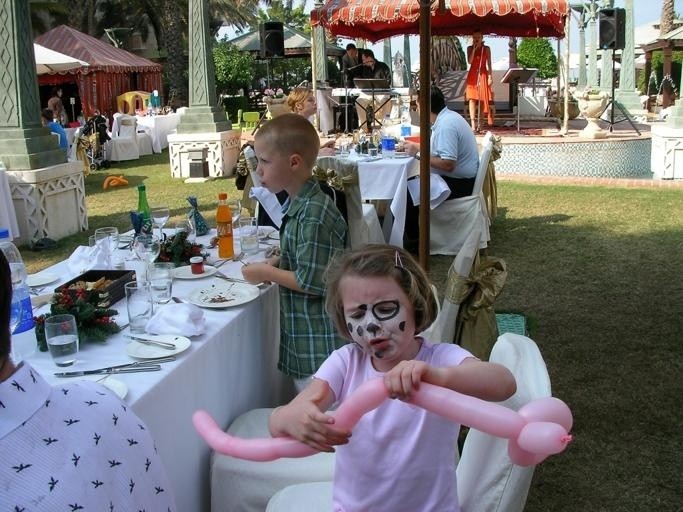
[71, 113, 111, 171]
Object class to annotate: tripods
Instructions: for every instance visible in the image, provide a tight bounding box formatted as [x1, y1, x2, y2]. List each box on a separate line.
[598, 47, 641, 135]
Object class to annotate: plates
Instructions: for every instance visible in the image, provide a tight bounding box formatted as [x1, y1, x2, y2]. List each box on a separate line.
[24, 273, 59, 287]
[93, 374, 127, 401]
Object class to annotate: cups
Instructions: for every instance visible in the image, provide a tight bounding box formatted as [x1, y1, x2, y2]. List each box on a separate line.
[339, 128, 407, 162]
[44, 217, 258, 368]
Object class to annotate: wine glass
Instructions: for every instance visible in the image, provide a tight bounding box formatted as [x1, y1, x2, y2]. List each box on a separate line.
[226, 198, 241, 223]
[147, 202, 169, 240]
[134, 106, 171, 114]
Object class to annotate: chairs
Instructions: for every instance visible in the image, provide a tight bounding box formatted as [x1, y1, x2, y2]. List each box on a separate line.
[204, 226, 509, 511]
[242, 146, 259, 227]
[260, 331, 553, 512]
[428, 130, 504, 257]
[231, 109, 243, 127]
[49, 105, 189, 162]
[241, 111, 260, 132]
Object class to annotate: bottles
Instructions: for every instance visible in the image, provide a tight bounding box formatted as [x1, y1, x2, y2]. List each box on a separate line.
[135, 183, 151, 216]
[216, 191, 234, 258]
[401, 106, 412, 137]
[0, 227, 38, 363]
[148, 102, 152, 116]
[380, 113, 395, 159]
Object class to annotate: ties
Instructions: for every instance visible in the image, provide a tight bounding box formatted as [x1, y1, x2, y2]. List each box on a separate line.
[353, 58, 358, 65]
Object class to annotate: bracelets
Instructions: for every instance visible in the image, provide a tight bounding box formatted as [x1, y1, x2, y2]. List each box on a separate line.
[416, 152, 420, 160]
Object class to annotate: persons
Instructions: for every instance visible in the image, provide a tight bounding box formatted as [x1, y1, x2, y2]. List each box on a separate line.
[287, 88, 336, 157]
[308, 43, 392, 131]
[241, 114, 350, 392]
[270, 245, 517, 511]
[0, 247, 176, 512]
[400, 85, 479, 242]
[466, 32, 493, 133]
[48, 87, 69, 127]
[42, 110, 68, 155]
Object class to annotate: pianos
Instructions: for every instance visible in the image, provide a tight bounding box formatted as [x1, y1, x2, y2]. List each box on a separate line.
[332, 86, 414, 96]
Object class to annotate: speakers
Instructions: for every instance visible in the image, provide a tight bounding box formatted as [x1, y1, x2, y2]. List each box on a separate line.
[259, 21, 284, 59]
[600, 7, 625, 48]
